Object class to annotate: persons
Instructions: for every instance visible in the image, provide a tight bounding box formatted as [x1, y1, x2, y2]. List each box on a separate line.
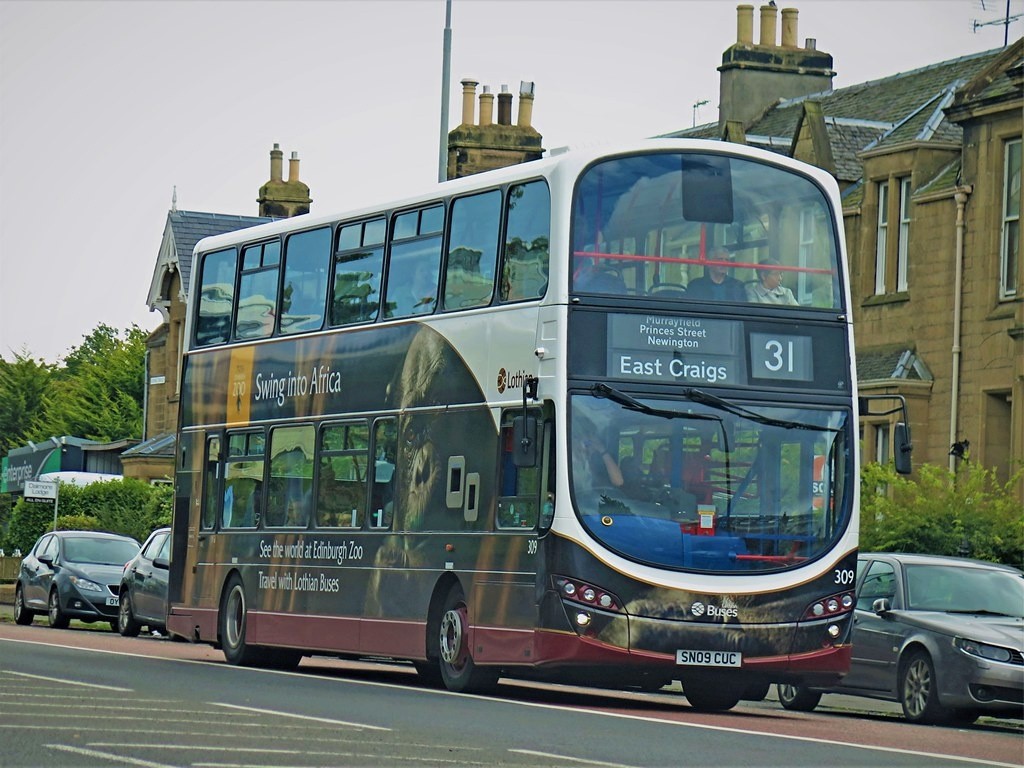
[389, 261, 438, 317]
[573, 415, 654, 502]
[685, 246, 748, 304]
[744, 260, 799, 306]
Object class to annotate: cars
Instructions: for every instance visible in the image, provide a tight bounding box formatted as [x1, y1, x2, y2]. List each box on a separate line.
[776, 552, 1023, 726]
[118, 527, 188, 642]
[14, 531, 142, 632]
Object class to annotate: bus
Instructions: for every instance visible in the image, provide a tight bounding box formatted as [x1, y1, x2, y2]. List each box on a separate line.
[166, 137, 915, 715]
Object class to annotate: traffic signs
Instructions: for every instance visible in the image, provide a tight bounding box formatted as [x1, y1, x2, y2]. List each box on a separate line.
[22, 480, 57, 503]
